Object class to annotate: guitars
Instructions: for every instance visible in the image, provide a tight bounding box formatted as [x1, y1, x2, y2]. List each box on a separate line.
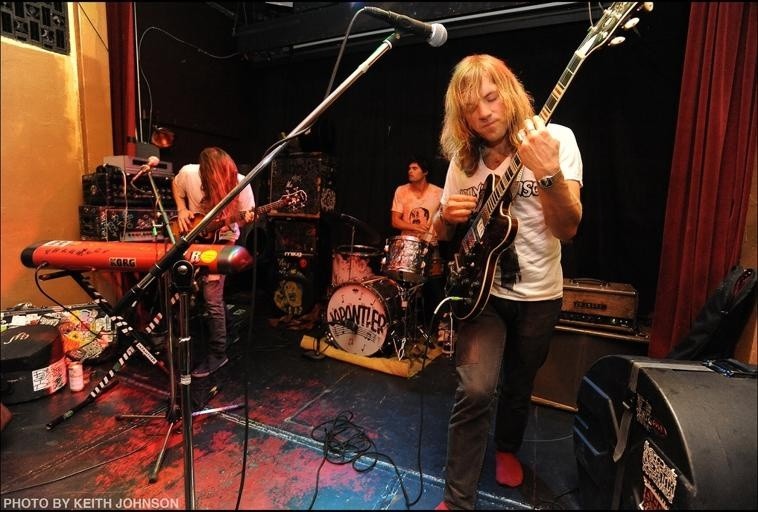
[442, 0, 657, 325]
[162, 185, 309, 244]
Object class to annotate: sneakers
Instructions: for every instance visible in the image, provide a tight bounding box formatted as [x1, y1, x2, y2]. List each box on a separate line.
[192, 352, 229, 378]
[442, 328, 457, 354]
[495, 450, 522, 487]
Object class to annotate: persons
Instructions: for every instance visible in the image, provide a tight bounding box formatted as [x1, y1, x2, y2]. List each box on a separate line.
[172, 149, 256, 378]
[389, 163, 448, 241]
[432, 55, 583, 511]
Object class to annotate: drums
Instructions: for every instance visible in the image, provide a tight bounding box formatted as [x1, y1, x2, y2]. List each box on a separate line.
[323, 273, 409, 358]
[385, 233, 436, 287]
[330, 242, 384, 287]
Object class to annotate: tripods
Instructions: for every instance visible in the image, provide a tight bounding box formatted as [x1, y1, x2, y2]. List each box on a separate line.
[114, 263, 246, 485]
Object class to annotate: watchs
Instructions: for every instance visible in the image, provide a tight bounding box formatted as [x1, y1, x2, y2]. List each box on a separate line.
[536, 170, 564, 190]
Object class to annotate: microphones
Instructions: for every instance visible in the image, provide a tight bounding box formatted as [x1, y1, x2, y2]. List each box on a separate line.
[130, 155, 160, 184]
[361, 6, 447, 48]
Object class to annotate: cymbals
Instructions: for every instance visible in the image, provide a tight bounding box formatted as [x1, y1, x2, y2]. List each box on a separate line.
[336, 211, 371, 231]
